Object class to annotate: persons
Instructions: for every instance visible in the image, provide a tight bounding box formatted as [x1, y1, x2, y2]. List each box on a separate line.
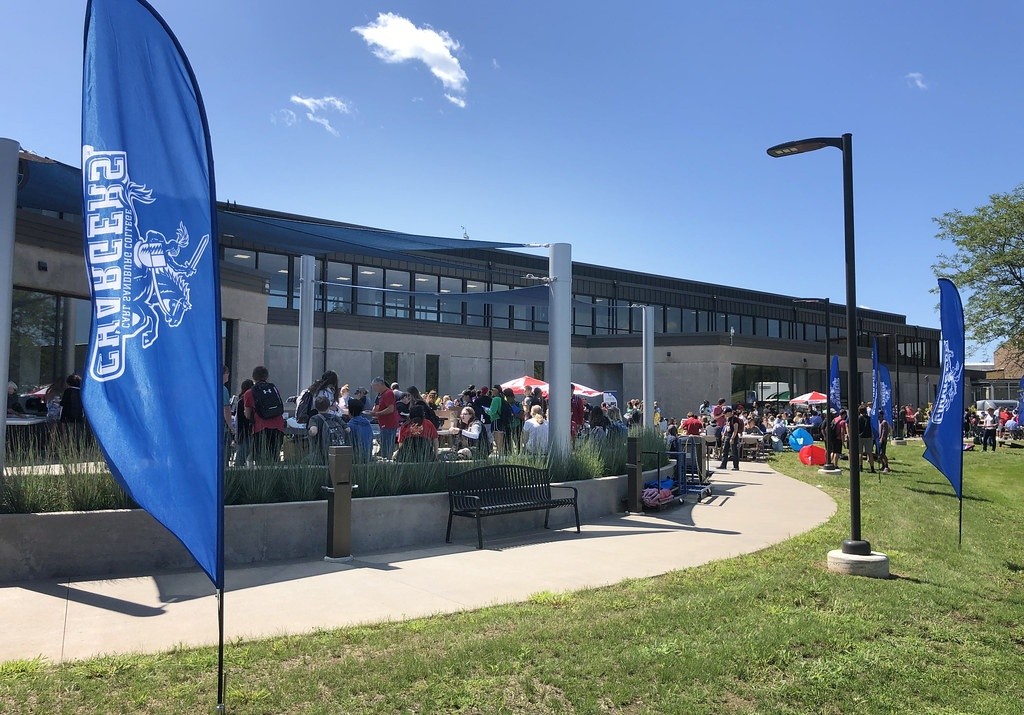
[653, 398, 822, 471]
[821, 401, 891, 474]
[5, 373, 94, 461]
[284, 370, 549, 465]
[757, 438, 764, 453]
[570, 383, 643, 449]
[763, 434, 771, 449]
[223, 366, 287, 467]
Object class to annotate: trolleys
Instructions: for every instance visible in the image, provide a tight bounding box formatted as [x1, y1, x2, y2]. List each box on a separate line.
[660, 452, 713, 503]
[698, 429, 714, 478]
[641, 451, 685, 512]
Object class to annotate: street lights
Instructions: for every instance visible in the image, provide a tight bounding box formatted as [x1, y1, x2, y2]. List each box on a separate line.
[792, 297, 836, 471]
[767, 131, 872, 556]
[905, 325, 920, 409]
[877, 333, 903, 440]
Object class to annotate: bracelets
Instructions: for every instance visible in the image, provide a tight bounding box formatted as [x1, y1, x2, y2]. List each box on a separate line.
[459, 429, 462, 435]
[895, 402, 1024, 453]
[881, 437, 883, 440]
[731, 432, 736, 439]
[438, 403, 439, 406]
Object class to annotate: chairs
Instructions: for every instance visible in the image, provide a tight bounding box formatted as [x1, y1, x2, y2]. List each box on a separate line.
[742, 439, 760, 462]
[705, 434, 720, 459]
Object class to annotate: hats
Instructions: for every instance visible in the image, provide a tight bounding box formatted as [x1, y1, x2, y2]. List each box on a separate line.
[830, 408, 837, 414]
[879, 410, 884, 415]
[723, 407, 732, 414]
[354, 386, 366, 395]
[493, 384, 506, 396]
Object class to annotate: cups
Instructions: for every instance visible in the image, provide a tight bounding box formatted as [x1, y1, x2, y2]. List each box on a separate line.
[743, 432, 747, 437]
[751, 433, 753, 436]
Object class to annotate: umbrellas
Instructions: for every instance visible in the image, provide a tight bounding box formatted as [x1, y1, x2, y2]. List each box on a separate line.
[499, 375, 604, 413]
[789, 391, 827, 426]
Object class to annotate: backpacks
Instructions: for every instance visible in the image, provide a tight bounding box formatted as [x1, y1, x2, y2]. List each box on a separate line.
[317, 413, 350, 459]
[732, 416, 744, 433]
[497, 396, 515, 424]
[251, 380, 285, 420]
[818, 419, 826, 440]
[296, 389, 313, 423]
[830, 419, 843, 440]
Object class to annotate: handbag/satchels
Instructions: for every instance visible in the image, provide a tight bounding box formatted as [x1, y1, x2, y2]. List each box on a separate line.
[993, 415, 998, 429]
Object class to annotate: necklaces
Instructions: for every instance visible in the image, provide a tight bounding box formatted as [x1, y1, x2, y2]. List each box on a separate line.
[378, 389, 388, 399]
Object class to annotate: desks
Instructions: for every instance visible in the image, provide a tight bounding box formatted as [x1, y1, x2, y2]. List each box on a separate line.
[741, 434, 764, 462]
[5, 417, 45, 452]
[785, 424, 815, 437]
[433, 429, 452, 455]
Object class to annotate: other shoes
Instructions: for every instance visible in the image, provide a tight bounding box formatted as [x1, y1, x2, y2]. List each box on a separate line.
[880, 466, 890, 475]
[716, 464, 726, 469]
[980, 449, 995, 452]
[732, 464, 739, 470]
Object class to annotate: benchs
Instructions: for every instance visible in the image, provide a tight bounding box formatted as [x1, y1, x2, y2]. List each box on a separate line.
[446, 465, 581, 549]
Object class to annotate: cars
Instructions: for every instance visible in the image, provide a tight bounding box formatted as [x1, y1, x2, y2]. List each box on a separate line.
[976, 400, 1019, 418]
